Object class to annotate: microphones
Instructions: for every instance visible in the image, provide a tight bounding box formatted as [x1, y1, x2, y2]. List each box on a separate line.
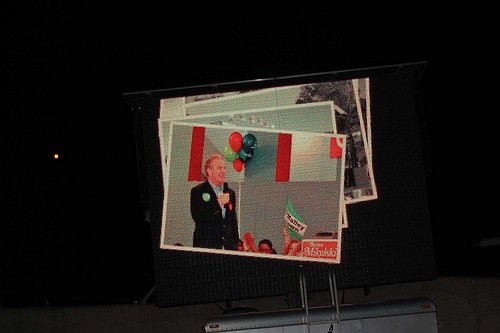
[223, 182, 229, 208]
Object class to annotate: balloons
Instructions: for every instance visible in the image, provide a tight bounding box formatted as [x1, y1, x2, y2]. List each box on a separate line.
[225, 132, 257, 172]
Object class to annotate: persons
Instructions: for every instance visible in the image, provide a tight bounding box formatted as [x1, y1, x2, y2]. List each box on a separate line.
[174, 225, 301, 256]
[190, 156, 239, 250]
